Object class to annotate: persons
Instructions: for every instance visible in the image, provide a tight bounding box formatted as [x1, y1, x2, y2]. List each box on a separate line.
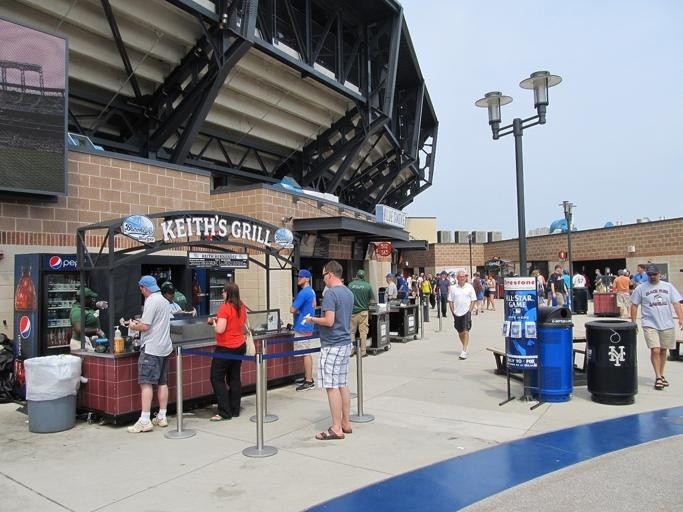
[160, 280, 196, 317]
[473, 272, 497, 314]
[384, 270, 455, 317]
[531, 264, 649, 319]
[123, 275, 173, 433]
[348, 270, 375, 357]
[156, 278, 187, 311]
[446, 270, 478, 359]
[628, 265, 683, 389]
[301, 259, 356, 440]
[68, 287, 107, 350]
[289, 269, 315, 392]
[207, 283, 246, 422]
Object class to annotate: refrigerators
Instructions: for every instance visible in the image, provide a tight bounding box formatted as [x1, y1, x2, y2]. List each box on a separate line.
[12, 252, 87, 393]
[191, 267, 235, 319]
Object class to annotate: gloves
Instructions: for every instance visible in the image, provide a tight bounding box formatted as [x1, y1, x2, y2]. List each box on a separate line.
[96, 328, 104, 337]
[96, 301, 109, 309]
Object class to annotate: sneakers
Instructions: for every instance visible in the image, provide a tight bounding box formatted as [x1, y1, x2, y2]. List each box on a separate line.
[127, 417, 153, 433]
[152, 412, 168, 427]
[296, 377, 314, 386]
[296, 381, 315, 391]
[459, 352, 467, 358]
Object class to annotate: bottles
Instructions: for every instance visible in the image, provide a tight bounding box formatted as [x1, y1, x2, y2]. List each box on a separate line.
[149, 266, 172, 281]
[130, 334, 141, 352]
[47, 275, 79, 290]
[48, 329, 71, 345]
[14, 256, 34, 394]
[113, 338, 124, 353]
[208, 273, 227, 315]
[191, 272, 201, 315]
[46, 309, 72, 326]
[47, 291, 76, 309]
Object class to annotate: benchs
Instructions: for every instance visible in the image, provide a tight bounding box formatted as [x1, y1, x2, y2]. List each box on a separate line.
[484, 341, 525, 382]
[571, 337, 588, 372]
[668, 339, 682, 363]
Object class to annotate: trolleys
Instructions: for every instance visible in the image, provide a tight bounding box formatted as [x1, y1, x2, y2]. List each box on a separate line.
[353, 311, 390, 356]
[389, 299, 418, 342]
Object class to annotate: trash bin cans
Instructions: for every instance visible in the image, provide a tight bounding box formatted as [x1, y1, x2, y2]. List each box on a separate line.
[584, 319, 638, 406]
[522, 306, 574, 403]
[572, 286, 588, 315]
[23, 354, 82, 433]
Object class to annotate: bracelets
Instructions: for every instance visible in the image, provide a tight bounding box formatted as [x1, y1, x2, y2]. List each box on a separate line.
[310, 317, 313, 322]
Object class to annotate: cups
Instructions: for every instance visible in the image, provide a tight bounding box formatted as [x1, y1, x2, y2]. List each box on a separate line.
[94, 338, 107, 353]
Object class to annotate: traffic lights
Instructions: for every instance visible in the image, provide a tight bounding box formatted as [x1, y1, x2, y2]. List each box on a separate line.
[557, 251, 567, 259]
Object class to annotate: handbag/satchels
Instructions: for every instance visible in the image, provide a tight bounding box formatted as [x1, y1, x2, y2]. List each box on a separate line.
[244, 331, 256, 357]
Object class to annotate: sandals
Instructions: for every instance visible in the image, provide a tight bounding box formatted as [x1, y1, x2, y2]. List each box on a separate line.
[655, 378, 663, 388]
[661, 376, 668, 386]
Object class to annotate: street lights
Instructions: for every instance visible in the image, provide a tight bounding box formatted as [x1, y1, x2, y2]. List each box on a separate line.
[558, 201, 577, 314]
[464, 232, 474, 280]
[474, 70, 561, 275]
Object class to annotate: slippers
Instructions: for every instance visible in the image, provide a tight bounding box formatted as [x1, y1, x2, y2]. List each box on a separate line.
[315, 426, 352, 440]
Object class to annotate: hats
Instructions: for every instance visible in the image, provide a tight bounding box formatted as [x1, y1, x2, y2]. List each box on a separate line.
[357, 270, 364, 276]
[77, 287, 97, 297]
[139, 275, 161, 292]
[163, 284, 176, 293]
[647, 266, 658, 273]
[294, 270, 311, 277]
